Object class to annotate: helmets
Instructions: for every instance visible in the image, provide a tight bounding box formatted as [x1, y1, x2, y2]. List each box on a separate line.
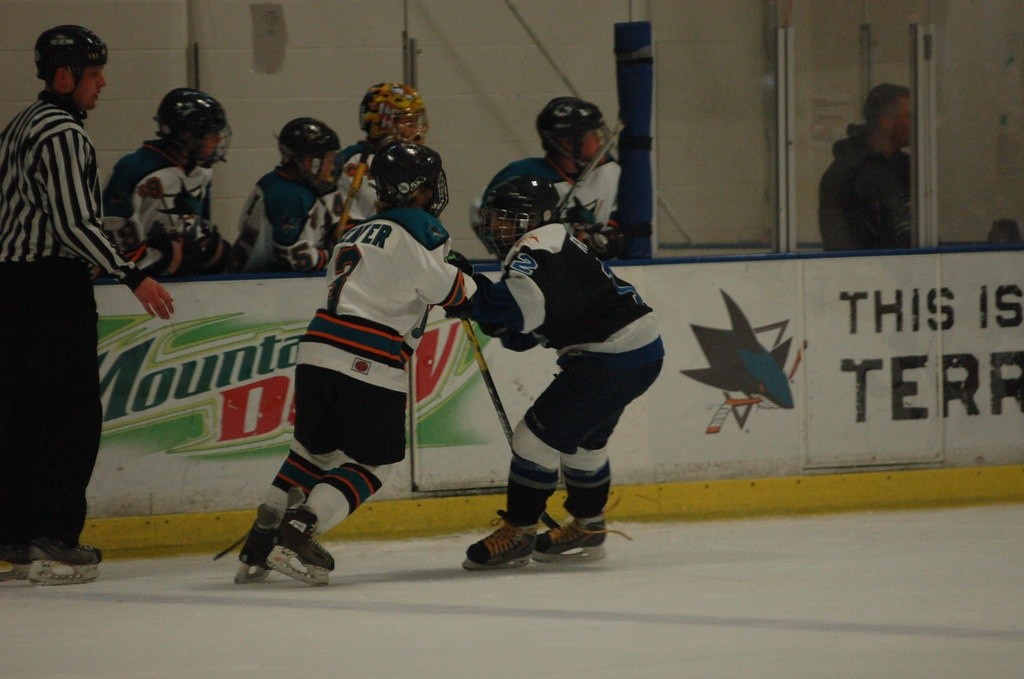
[369, 142, 449, 218]
[534, 96, 606, 136]
[475, 174, 561, 256]
[359, 83, 429, 145]
[33, 24, 107, 79]
[152, 87, 233, 161]
[278, 117, 341, 154]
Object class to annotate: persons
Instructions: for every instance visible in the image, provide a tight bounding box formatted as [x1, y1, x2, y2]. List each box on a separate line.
[335, 82, 430, 230]
[99, 88, 235, 278]
[0, 25, 176, 585]
[461, 175, 668, 571]
[817, 84, 915, 251]
[238, 116, 340, 273]
[230, 141, 486, 586]
[534, 97, 653, 261]
[988, 221, 1021, 244]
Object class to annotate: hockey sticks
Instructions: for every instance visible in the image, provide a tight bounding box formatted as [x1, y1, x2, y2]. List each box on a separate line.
[560, 122, 627, 222]
[212, 322, 423, 562]
[462, 319, 562, 532]
[329, 161, 370, 262]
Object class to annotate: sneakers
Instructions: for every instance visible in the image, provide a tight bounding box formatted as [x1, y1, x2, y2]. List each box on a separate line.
[461, 509, 538, 571]
[28, 538, 102, 586]
[530, 513, 634, 564]
[234, 521, 274, 585]
[0, 545, 31, 582]
[265, 504, 335, 585]
